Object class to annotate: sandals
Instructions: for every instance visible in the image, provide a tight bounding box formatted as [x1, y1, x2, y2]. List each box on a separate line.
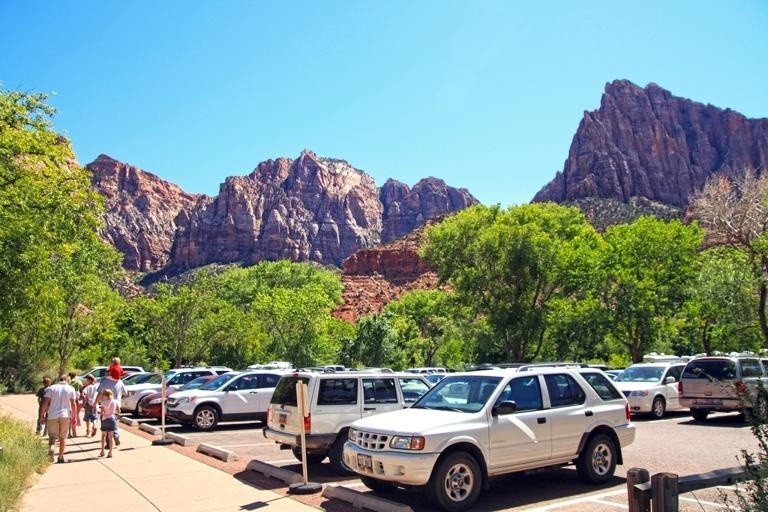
[35, 428, 80, 440]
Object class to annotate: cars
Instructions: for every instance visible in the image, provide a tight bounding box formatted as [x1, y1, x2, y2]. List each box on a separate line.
[138, 375, 218, 423]
[600, 361, 688, 420]
[75, 360, 628, 419]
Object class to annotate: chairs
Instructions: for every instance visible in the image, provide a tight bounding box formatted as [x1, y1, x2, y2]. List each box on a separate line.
[365, 388, 387, 399]
[478, 384, 536, 408]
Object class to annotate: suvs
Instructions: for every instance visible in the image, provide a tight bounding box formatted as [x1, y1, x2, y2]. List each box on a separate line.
[262, 366, 452, 477]
[340, 361, 635, 512]
[165, 369, 293, 432]
[678, 355, 768, 425]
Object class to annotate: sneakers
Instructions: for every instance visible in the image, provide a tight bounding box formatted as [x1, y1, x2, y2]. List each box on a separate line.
[46, 452, 73, 463]
[85, 428, 121, 458]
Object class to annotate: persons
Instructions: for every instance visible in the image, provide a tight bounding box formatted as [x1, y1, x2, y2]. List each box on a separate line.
[37, 357, 128, 463]
